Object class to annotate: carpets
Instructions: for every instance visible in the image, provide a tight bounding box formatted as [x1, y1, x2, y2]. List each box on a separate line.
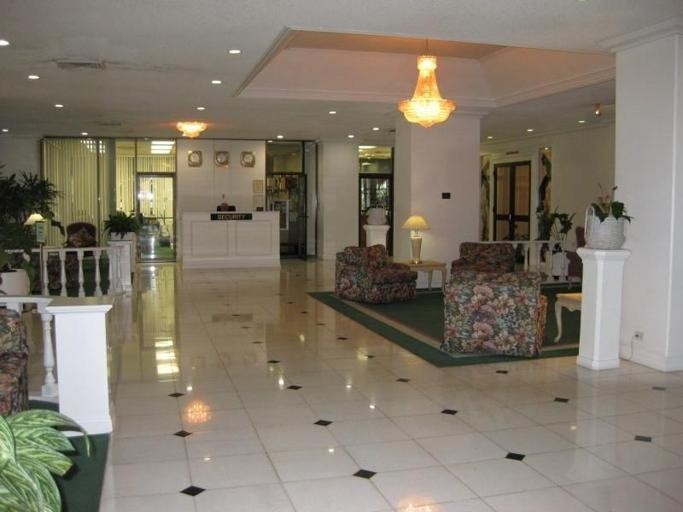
[305, 276, 581, 367]
[24, 400, 111, 511]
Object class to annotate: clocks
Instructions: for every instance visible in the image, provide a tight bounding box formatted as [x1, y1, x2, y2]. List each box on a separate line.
[240, 151, 255, 167]
[213, 148, 230, 166]
[186, 150, 202, 167]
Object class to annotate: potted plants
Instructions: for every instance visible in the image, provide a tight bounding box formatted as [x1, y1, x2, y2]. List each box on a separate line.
[533, 202, 575, 279]
[101, 210, 139, 273]
[0, 222, 38, 298]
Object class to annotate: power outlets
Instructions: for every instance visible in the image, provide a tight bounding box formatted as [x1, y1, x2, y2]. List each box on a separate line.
[633, 330, 644, 343]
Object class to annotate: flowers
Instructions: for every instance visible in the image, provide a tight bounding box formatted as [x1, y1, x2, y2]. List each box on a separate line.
[586, 181, 632, 223]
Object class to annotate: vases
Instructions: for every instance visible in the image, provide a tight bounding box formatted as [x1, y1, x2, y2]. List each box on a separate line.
[584, 215, 625, 250]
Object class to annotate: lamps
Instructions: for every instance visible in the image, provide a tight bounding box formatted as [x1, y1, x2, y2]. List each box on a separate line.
[175, 121, 208, 138]
[22, 213, 44, 227]
[400, 215, 430, 263]
[398, 38, 455, 129]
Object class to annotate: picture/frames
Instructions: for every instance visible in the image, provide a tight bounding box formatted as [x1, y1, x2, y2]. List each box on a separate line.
[252, 178, 264, 194]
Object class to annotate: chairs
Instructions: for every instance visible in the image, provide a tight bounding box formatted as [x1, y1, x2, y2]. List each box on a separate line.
[10, 237, 79, 291]
[338, 243, 417, 305]
[66, 221, 97, 257]
[440, 270, 546, 360]
[0, 308, 29, 419]
[563, 225, 585, 291]
[451, 242, 518, 275]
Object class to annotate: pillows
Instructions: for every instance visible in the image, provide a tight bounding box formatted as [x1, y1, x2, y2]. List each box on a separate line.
[67, 226, 95, 248]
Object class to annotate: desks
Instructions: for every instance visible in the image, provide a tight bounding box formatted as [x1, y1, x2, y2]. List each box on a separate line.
[552, 292, 580, 343]
[401, 258, 447, 297]
